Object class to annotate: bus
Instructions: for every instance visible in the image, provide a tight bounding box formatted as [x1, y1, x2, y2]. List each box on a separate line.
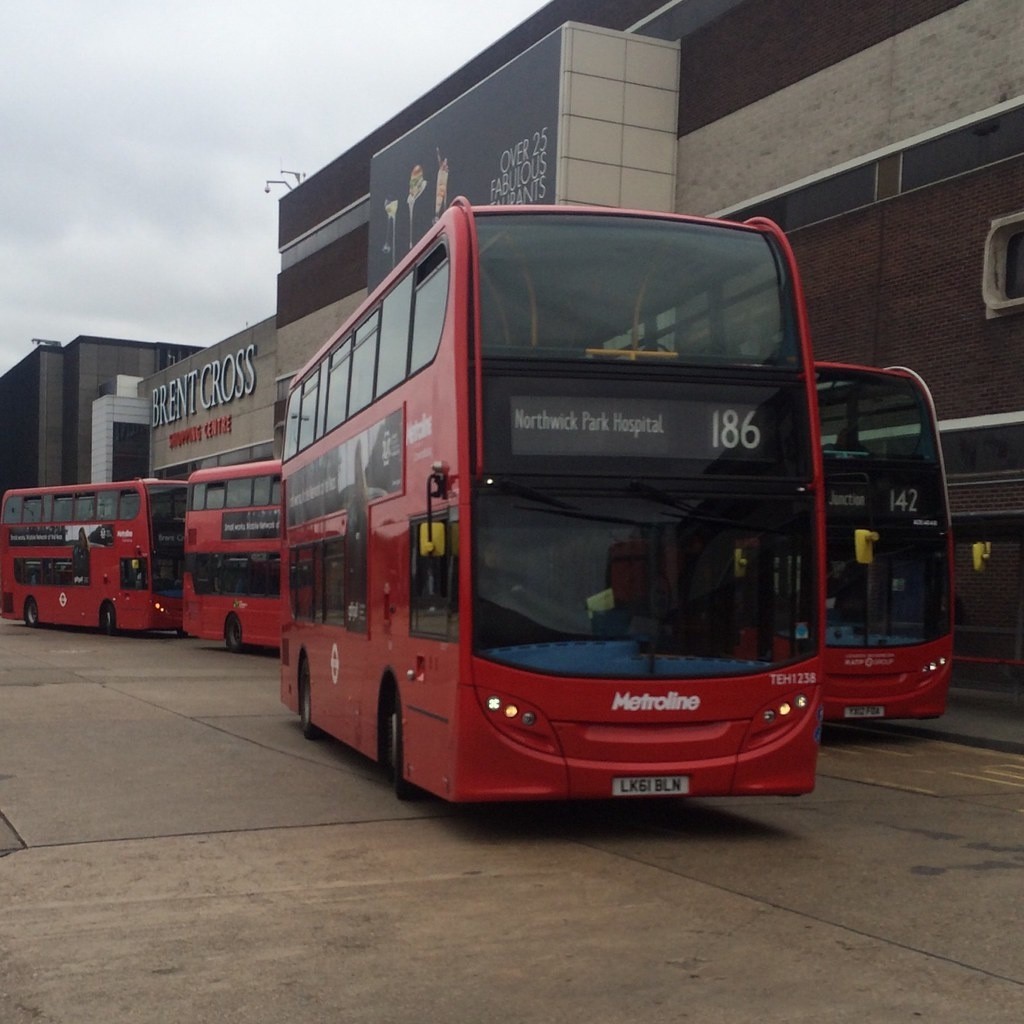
[734, 361, 992, 724]
[182, 460, 363, 655]
[280, 194, 882, 806]
[0, 476, 189, 637]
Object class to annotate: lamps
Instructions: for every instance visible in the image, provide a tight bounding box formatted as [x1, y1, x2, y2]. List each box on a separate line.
[264, 178, 293, 195]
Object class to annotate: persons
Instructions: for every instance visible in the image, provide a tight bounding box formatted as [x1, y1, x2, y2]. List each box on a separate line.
[835, 419, 858, 443]
